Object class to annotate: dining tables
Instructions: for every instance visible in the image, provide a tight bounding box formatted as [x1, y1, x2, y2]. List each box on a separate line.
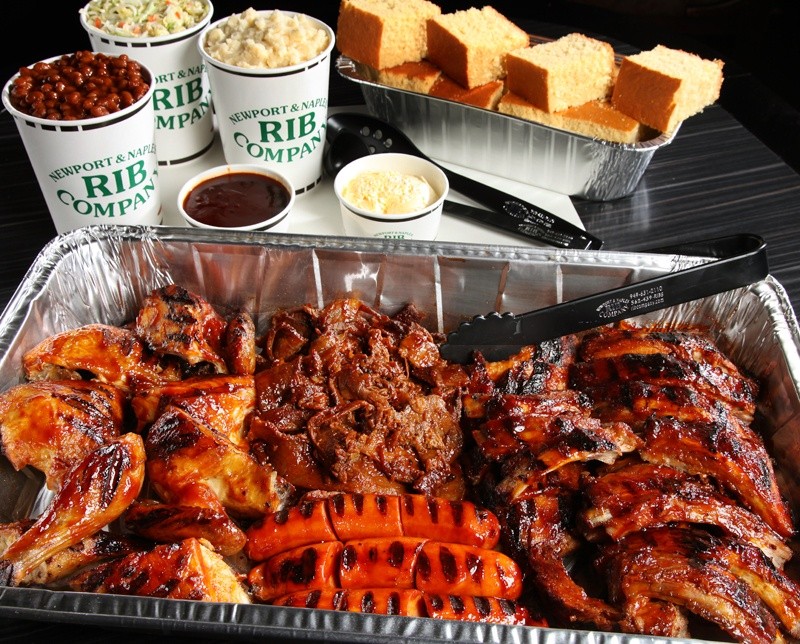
[0, 33, 800, 644]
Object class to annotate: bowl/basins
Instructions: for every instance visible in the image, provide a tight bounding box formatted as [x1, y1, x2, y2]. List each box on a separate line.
[335, 32, 684, 201]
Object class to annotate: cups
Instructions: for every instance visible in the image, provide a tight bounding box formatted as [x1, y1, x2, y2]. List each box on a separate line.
[197, 9, 336, 198]
[176, 163, 295, 233]
[78, 0, 215, 169]
[2, 52, 166, 235]
[333, 152, 449, 241]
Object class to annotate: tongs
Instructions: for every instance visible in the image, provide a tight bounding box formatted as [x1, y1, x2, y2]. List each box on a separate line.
[437, 231, 770, 364]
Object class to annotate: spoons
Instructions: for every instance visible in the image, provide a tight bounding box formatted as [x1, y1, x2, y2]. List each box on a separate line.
[323, 111, 605, 251]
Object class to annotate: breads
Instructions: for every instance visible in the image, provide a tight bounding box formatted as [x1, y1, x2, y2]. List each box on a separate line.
[332, 0, 724, 143]
